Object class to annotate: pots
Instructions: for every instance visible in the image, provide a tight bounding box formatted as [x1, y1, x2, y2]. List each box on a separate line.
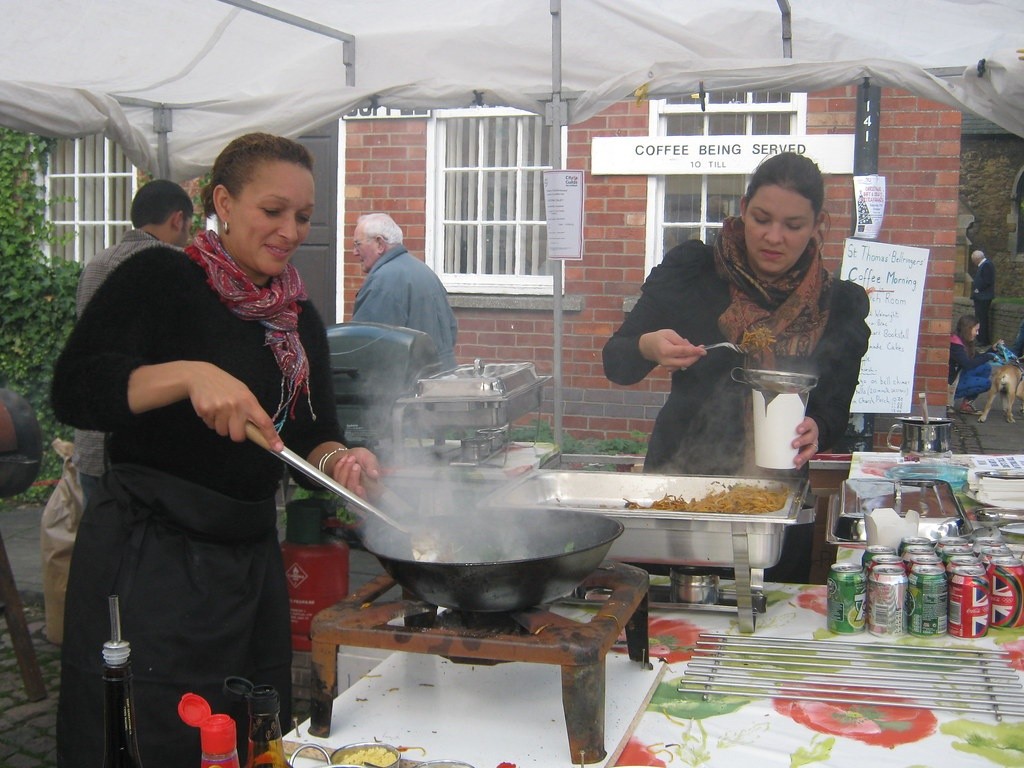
[356, 508, 626, 614]
[887, 415, 954, 457]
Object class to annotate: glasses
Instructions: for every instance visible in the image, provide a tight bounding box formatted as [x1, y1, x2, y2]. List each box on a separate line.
[354, 237, 375, 249]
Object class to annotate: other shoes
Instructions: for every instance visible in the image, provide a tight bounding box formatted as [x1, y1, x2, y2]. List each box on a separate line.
[976, 342, 989, 347]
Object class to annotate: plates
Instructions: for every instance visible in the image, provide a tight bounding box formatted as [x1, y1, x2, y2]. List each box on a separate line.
[883, 463, 1024, 536]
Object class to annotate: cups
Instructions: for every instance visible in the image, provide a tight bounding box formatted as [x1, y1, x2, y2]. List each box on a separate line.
[751, 388, 810, 470]
[461, 423, 510, 463]
[414, 759, 475, 768]
[331, 743, 401, 768]
[670, 565, 720, 605]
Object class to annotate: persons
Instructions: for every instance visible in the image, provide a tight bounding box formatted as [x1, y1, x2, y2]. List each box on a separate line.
[971, 250, 996, 347]
[352, 213, 457, 443]
[72, 179, 209, 519]
[602, 153, 871, 585]
[50, 132, 380, 768]
[947, 315, 1005, 415]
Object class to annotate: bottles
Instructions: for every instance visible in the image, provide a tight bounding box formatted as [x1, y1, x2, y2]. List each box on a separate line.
[223, 676, 294, 768]
[178, 692, 243, 768]
[100, 661, 145, 768]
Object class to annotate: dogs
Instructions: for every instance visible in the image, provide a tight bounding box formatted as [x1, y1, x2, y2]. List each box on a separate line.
[978, 364, 1024, 424]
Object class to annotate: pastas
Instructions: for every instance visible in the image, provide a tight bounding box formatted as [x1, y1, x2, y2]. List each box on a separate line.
[624, 483, 792, 515]
[740, 326, 777, 353]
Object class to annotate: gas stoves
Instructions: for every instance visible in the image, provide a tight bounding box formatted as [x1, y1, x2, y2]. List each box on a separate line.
[307, 559, 654, 765]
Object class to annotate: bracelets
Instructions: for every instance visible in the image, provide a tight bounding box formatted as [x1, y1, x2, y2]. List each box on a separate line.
[318, 447, 348, 474]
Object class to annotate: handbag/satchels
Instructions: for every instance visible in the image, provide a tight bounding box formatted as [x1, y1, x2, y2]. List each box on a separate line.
[947, 375, 959, 407]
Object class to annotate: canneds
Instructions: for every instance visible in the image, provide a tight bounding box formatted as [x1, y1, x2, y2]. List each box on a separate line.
[828, 563, 867, 635]
[861, 537, 1024, 639]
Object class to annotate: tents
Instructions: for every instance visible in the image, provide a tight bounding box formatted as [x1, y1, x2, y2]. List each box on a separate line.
[0, 0, 1024, 451]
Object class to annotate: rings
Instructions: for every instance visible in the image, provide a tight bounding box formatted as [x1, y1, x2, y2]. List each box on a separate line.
[810, 444, 818, 453]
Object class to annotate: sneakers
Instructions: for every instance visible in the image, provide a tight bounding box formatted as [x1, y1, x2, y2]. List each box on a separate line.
[959, 399, 982, 415]
